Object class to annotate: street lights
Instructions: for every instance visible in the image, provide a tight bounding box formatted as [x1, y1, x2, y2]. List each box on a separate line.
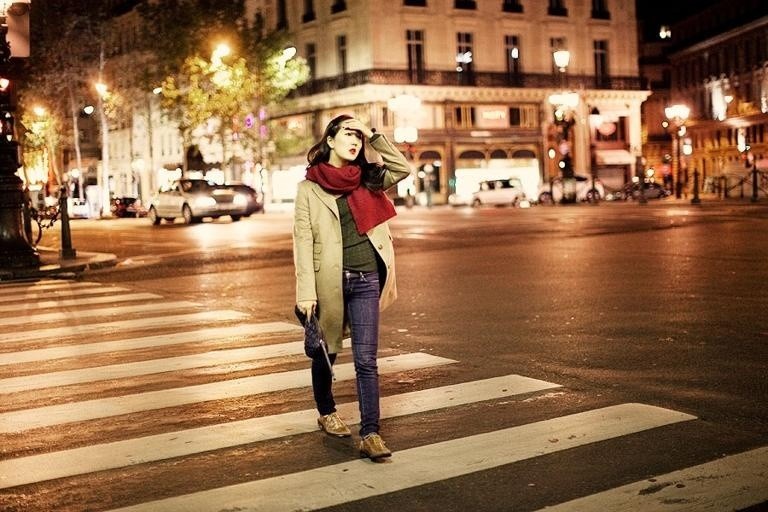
[665, 105, 689, 199]
[548, 51, 580, 204]
[388, 95, 421, 209]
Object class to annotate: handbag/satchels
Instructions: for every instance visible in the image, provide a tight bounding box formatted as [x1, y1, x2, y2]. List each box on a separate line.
[294, 304, 329, 360]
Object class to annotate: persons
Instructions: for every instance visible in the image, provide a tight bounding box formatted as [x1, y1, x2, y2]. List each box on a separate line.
[294, 115, 411, 459]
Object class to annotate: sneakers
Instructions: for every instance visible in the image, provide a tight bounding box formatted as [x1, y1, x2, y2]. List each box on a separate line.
[359, 432, 392, 459]
[318, 410, 352, 436]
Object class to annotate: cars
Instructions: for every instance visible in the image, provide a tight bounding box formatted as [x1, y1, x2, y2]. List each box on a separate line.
[530, 173, 605, 205]
[147, 178, 263, 225]
[613, 182, 669, 201]
[109, 199, 144, 217]
[447, 180, 526, 207]
